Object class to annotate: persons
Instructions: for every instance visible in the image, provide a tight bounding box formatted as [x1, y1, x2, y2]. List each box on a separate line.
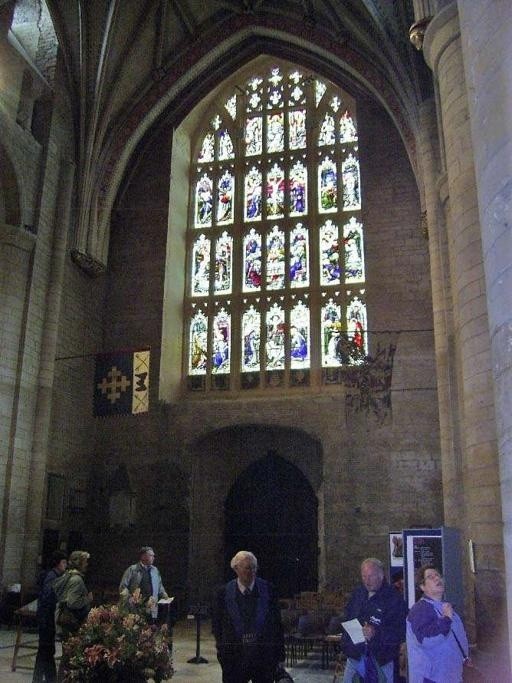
[52, 550, 94, 683]
[403, 562, 473, 683]
[118, 545, 170, 626]
[32, 550, 68, 682]
[338, 556, 410, 683]
[210, 550, 287, 682]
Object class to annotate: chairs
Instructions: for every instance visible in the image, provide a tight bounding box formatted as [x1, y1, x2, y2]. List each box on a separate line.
[280, 591, 347, 669]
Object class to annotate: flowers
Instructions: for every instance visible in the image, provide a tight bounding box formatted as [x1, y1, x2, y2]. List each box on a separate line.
[56, 599, 170, 682]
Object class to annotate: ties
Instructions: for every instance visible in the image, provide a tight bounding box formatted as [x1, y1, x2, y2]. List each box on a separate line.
[244, 586, 256, 634]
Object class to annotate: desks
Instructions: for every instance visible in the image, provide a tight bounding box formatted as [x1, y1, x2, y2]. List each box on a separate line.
[11, 598, 39, 672]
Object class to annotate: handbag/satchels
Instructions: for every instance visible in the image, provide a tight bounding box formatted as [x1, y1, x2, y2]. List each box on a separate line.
[56, 603, 87, 633]
[274, 666, 294, 683]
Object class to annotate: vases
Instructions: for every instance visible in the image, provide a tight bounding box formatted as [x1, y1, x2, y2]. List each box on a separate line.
[93, 660, 146, 682]
